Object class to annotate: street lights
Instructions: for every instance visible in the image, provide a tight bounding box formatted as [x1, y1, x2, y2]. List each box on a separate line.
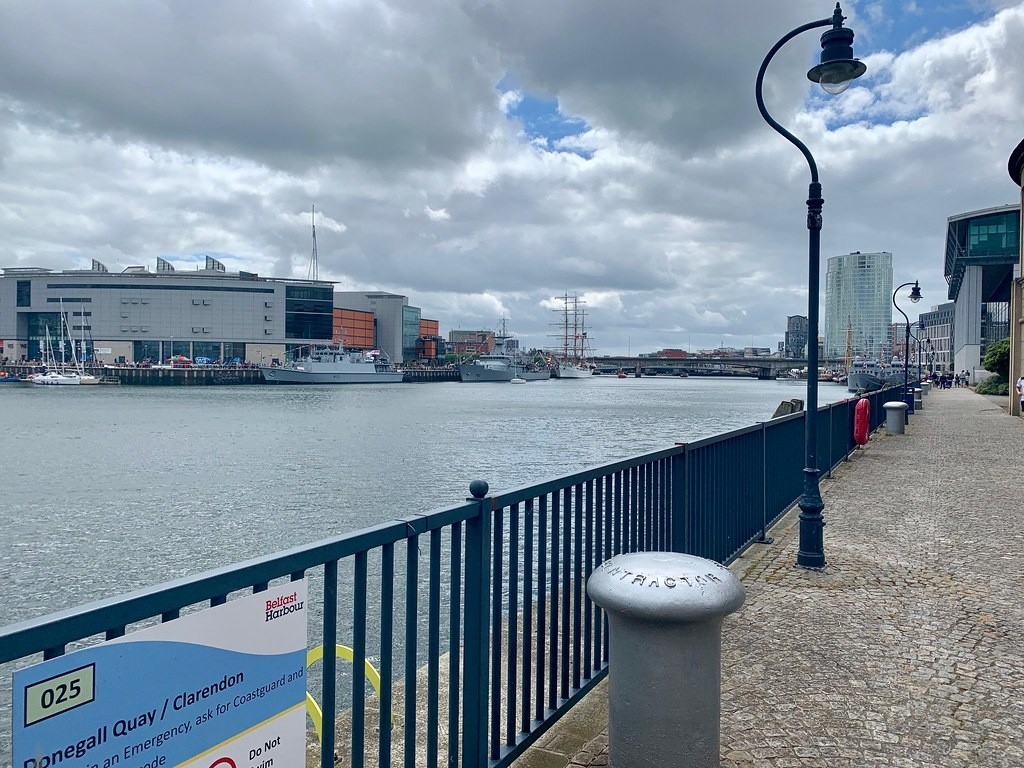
[909, 315, 936, 382]
[751, 2, 869, 567]
[892, 279, 925, 424]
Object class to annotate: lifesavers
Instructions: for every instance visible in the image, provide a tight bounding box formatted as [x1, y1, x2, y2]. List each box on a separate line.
[853, 399, 871, 445]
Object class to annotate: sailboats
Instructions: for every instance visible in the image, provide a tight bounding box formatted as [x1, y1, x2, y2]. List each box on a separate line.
[510, 337, 527, 383]
[541, 289, 598, 378]
[0, 294, 106, 385]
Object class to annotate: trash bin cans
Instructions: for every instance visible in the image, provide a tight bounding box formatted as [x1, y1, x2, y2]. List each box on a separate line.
[900, 387, 915, 414]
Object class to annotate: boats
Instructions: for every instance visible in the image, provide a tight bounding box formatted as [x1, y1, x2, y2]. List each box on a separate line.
[644, 368, 658, 376]
[593, 371, 602, 375]
[456, 310, 552, 382]
[680, 371, 689, 377]
[257, 325, 405, 384]
[617, 373, 628, 378]
[846, 354, 887, 392]
[802, 364, 847, 384]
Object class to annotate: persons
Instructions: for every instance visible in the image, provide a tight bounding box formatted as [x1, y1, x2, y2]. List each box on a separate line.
[925, 368, 970, 390]
[1016, 376, 1024, 412]
